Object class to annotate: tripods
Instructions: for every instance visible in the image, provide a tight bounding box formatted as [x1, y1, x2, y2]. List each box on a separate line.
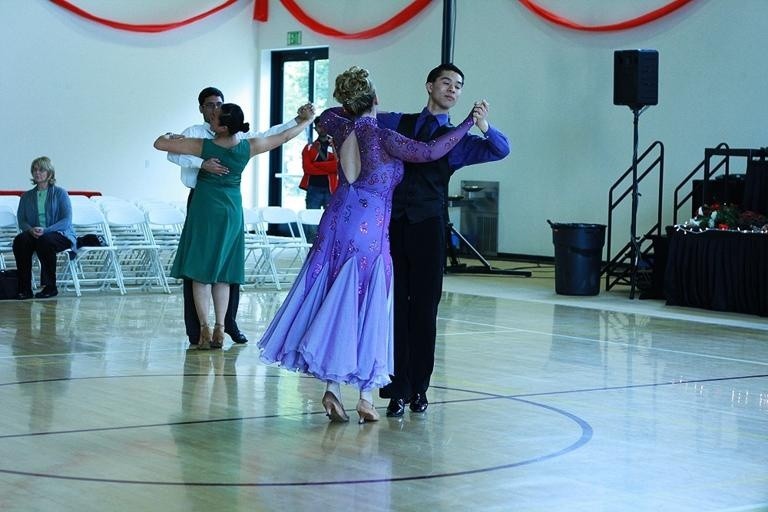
[600, 112, 653, 299]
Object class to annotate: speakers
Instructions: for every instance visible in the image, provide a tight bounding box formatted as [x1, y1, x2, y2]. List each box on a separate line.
[614, 49, 659, 105]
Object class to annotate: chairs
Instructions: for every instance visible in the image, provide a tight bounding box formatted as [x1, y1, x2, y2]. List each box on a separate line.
[629, 231, 656, 299]
[0, 190, 326, 295]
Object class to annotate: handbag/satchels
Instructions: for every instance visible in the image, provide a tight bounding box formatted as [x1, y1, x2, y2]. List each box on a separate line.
[0, 269, 22, 300]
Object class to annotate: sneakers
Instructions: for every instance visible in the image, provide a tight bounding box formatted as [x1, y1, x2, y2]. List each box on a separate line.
[16, 289, 34, 300]
[35, 287, 58, 299]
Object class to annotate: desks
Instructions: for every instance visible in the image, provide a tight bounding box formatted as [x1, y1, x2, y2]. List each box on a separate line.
[663, 224, 768, 318]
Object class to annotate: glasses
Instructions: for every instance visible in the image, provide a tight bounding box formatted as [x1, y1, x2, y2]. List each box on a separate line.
[203, 101, 222, 109]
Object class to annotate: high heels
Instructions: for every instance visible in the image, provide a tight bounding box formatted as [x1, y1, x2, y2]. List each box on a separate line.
[355, 399, 380, 424]
[198, 324, 211, 350]
[212, 323, 224, 348]
[322, 392, 349, 424]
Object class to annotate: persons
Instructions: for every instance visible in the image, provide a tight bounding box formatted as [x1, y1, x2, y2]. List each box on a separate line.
[298, 115, 338, 245]
[257, 66, 486, 425]
[168, 87, 316, 344]
[155, 104, 316, 351]
[12, 157, 77, 299]
[375, 63, 510, 416]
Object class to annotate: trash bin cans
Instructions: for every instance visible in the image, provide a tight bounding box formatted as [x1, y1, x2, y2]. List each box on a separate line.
[549, 223, 607, 296]
[460, 180, 499, 258]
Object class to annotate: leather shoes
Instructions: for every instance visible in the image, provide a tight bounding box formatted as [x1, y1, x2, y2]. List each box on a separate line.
[409, 390, 429, 414]
[225, 324, 247, 344]
[386, 398, 405, 418]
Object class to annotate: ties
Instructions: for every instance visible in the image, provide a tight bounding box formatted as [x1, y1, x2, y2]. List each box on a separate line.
[417, 116, 434, 166]
